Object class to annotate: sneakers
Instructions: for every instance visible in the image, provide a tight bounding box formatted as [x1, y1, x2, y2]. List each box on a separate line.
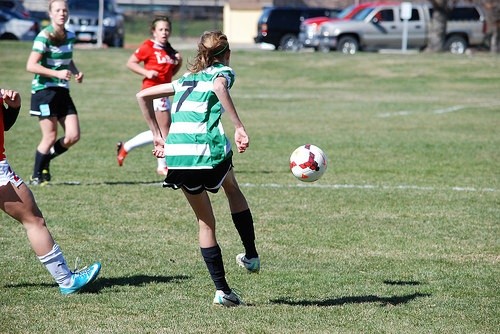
[58, 262, 101, 294]
[29, 176, 47, 187]
[43, 170, 50, 180]
[116, 142, 128, 166]
[157, 166, 168, 175]
[236, 253, 260, 272]
[213, 288, 247, 307]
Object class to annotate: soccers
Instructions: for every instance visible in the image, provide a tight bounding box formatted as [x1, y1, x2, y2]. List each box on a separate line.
[290, 144, 328, 183]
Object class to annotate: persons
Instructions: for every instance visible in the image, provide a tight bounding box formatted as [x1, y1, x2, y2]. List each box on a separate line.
[136, 30, 260, 306]
[0, 88, 102, 295]
[26, 0, 84, 185]
[479, 0, 500, 52]
[115, 15, 182, 177]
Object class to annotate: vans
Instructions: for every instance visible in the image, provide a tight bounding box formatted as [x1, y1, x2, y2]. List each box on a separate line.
[254, 6, 343, 52]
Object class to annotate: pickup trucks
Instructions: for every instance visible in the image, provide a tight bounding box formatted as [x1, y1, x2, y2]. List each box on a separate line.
[313, 4, 488, 55]
[299, 0, 409, 52]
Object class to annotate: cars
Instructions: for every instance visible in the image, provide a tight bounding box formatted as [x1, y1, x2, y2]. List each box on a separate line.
[64, 0, 125, 48]
[0, 9, 50, 40]
